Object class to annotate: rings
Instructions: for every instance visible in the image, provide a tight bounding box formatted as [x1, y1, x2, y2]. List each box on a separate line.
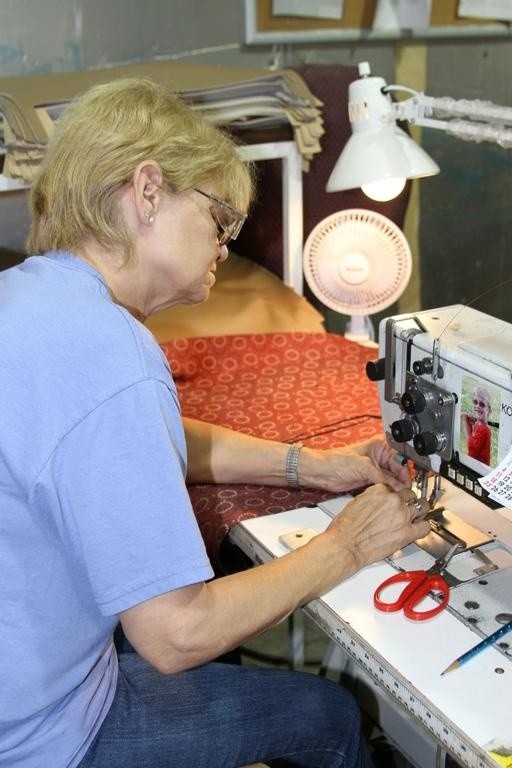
[408, 500, 422, 514]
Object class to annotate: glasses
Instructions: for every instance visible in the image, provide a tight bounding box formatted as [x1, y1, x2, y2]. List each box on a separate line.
[166, 167, 251, 247]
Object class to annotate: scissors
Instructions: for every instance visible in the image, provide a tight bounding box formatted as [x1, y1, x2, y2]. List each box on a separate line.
[372, 542, 460, 622]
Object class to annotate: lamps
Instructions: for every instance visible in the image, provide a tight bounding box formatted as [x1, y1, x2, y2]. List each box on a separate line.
[326, 58, 510, 202]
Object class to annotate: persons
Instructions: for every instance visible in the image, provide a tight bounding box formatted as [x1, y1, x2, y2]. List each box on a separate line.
[0, 72, 437, 766]
[458, 386, 493, 466]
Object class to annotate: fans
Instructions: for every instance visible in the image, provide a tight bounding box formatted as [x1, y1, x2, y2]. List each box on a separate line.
[302, 206, 413, 350]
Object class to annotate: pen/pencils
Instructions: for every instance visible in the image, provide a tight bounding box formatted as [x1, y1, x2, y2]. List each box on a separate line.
[441, 620, 512, 677]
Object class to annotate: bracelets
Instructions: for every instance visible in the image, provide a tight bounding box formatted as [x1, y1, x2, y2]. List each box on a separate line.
[286, 441, 305, 490]
